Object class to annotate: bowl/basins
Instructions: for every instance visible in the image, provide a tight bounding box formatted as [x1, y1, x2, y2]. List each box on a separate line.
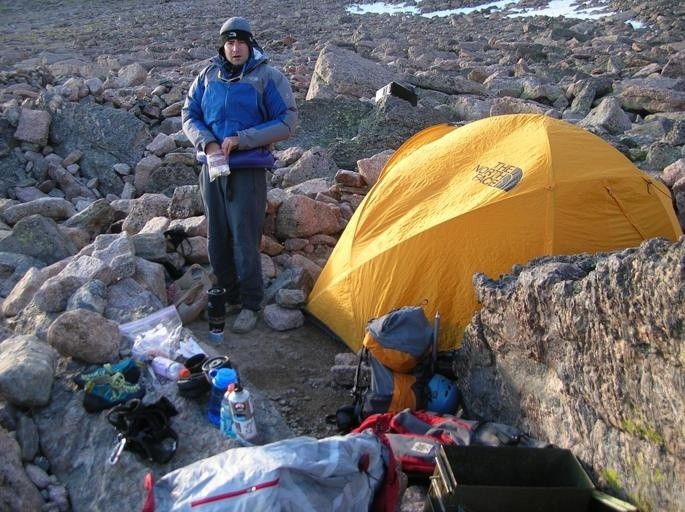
[185, 354, 207, 374]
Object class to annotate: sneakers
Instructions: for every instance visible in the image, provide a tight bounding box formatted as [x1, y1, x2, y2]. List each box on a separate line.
[230, 309, 259, 334]
[74, 358, 146, 412]
[203, 302, 243, 320]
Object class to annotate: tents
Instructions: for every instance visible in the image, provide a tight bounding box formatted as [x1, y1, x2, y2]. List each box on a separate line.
[302, 113, 682, 359]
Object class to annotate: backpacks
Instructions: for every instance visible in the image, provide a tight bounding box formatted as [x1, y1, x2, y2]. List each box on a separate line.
[333, 306, 435, 435]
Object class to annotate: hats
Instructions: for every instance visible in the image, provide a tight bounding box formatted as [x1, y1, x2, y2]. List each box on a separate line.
[220, 16, 253, 53]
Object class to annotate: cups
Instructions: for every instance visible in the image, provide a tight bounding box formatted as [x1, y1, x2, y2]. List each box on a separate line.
[203, 355, 233, 385]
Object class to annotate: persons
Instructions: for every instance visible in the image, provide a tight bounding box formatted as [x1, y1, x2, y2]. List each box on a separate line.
[181, 17, 297, 334]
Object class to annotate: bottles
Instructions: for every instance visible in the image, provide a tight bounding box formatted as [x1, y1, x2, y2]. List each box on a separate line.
[151, 356, 191, 381]
[208, 369, 259, 447]
[207, 287, 227, 334]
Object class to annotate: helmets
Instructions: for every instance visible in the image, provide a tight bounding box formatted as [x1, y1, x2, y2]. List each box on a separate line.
[424, 373, 459, 415]
